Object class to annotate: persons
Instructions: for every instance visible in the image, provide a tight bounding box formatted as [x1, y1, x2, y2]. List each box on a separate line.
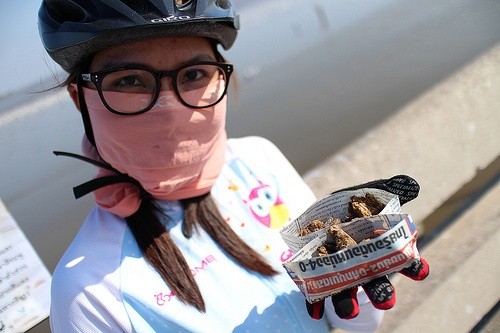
[37, 0, 430, 333]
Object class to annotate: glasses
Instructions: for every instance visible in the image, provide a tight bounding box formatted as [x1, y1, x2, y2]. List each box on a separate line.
[80, 61, 233, 116]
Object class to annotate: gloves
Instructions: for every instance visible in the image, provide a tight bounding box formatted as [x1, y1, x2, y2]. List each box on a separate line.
[306, 175, 430, 320]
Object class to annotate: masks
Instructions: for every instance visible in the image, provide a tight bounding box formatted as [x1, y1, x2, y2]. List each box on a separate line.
[82, 79, 227, 200]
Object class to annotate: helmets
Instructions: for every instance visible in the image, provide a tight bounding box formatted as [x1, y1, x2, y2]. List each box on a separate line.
[38, 0, 241, 51]
